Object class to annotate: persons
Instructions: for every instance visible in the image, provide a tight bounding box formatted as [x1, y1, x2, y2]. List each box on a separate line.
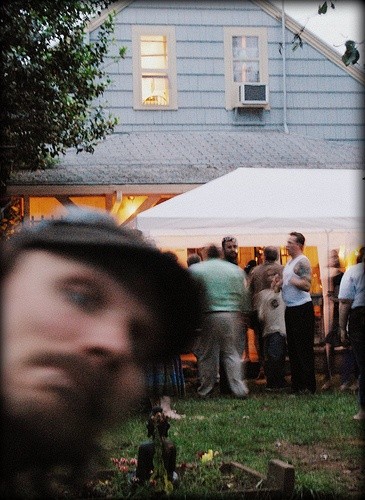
[0, 219, 211, 500]
[160, 233, 365, 421]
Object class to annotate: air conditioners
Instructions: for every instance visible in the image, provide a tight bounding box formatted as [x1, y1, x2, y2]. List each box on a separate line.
[238, 83, 269, 105]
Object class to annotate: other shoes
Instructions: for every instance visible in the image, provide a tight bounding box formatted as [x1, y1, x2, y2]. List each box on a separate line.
[155, 383, 365, 421]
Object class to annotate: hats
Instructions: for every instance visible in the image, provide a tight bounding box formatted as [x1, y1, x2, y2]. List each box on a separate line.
[12, 207, 207, 354]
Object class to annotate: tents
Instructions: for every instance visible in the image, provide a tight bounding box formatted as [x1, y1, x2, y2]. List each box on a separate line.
[122, 167, 365, 246]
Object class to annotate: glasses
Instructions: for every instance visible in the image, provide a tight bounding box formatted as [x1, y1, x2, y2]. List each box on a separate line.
[223, 236, 237, 242]
